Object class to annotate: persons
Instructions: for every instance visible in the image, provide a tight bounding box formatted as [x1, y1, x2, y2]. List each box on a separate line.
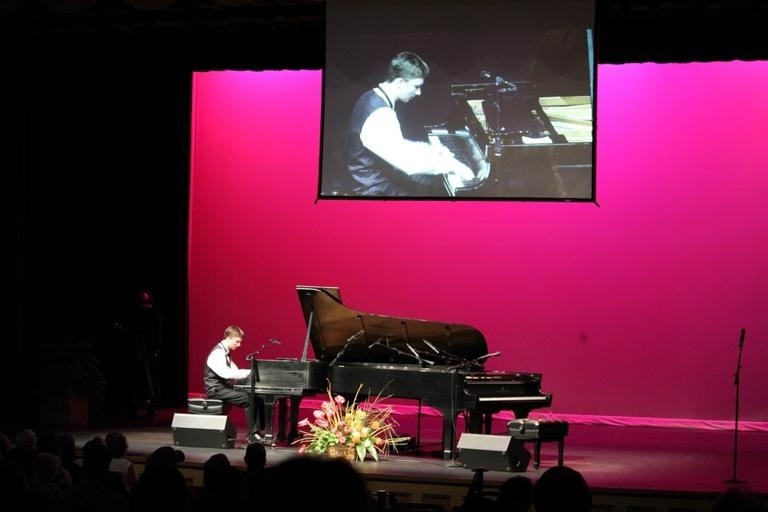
[462, 496, 498, 510]
[498, 476, 533, 511]
[534, 466, 593, 511]
[204, 326, 265, 444]
[345, 50, 476, 196]
[2, 426, 381, 510]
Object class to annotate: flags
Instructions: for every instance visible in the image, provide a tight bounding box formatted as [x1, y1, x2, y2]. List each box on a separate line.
[326, 444, 356, 463]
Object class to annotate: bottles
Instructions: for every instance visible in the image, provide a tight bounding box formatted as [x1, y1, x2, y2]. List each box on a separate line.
[147, 446, 185, 466]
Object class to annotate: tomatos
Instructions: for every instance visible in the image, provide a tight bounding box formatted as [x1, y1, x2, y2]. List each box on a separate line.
[234, 287, 550, 459]
[423, 66, 592, 199]
[455, 370, 552, 411]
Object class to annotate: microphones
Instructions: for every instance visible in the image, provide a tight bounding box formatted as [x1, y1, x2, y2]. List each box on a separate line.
[507, 418, 569, 470]
[188, 397, 230, 415]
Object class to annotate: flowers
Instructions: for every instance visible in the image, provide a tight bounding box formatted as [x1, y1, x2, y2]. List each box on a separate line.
[347, 329, 365, 341]
[738, 327, 746, 346]
[479, 351, 502, 359]
[268, 338, 281, 345]
[479, 70, 516, 87]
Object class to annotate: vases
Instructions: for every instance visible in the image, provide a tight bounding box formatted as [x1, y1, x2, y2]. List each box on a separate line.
[289, 376, 403, 463]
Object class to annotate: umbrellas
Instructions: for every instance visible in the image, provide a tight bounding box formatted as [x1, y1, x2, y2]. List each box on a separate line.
[245, 432, 264, 444]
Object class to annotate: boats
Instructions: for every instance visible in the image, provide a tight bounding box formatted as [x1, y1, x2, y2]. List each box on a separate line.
[170, 412, 238, 449]
[456, 432, 531, 473]
